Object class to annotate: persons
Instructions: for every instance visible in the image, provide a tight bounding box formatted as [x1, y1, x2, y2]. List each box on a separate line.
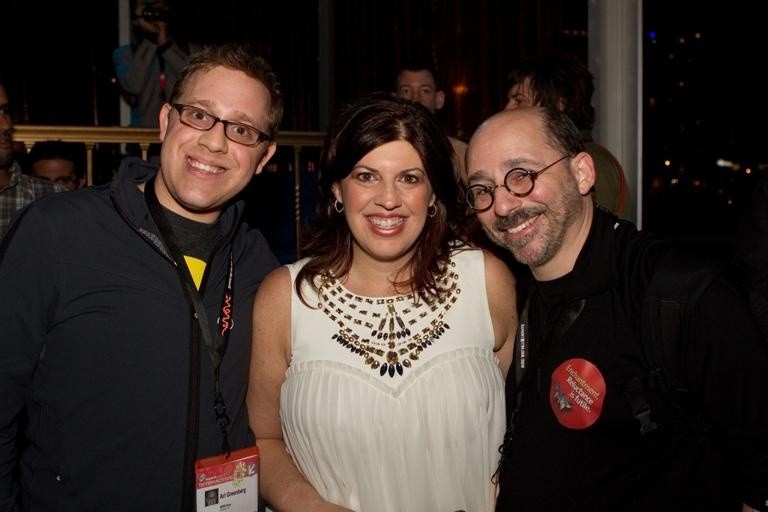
[501, 61, 638, 227]
[111, 0, 187, 158]
[1, 37, 281, 510]
[242, 99, 518, 512]
[0, 89, 70, 241]
[26, 136, 88, 196]
[462, 105, 766, 512]
[383, 60, 470, 188]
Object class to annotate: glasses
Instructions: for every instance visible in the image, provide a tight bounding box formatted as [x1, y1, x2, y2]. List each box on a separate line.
[467, 154, 568, 211]
[170, 103, 275, 148]
